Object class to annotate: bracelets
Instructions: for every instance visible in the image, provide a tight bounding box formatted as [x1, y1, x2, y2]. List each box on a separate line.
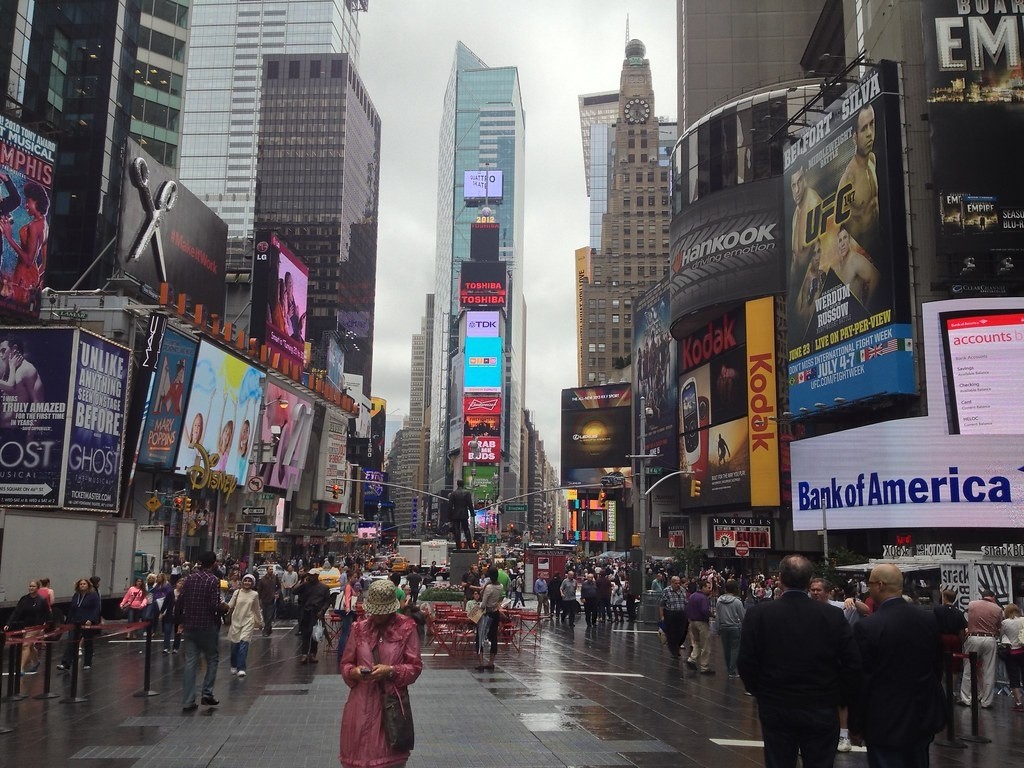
[390, 666, 393, 678]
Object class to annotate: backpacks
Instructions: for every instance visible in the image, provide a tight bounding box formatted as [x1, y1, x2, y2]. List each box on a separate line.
[613, 585, 623, 605]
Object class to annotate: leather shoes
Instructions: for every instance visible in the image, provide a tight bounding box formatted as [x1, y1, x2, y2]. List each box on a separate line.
[201, 696, 219, 705]
[183, 704, 198, 714]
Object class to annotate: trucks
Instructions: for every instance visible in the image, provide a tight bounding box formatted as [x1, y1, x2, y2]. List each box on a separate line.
[421, 539, 460, 575]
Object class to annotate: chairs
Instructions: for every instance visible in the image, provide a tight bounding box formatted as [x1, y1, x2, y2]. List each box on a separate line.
[422, 602, 550, 657]
[326, 601, 364, 652]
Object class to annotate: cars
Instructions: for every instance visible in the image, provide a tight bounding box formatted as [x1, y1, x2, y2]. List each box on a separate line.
[590, 551, 631, 565]
[314, 566, 343, 589]
[256, 563, 287, 581]
[367, 556, 387, 570]
[388, 556, 411, 573]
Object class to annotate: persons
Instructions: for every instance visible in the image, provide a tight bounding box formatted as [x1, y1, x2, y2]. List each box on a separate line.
[0, 174, 50, 300]
[535, 552, 633, 627]
[638, 329, 673, 397]
[4, 576, 104, 677]
[337, 580, 423, 768]
[463, 553, 524, 670]
[645, 555, 1024, 768]
[184, 388, 257, 483]
[120, 543, 439, 676]
[790, 103, 882, 308]
[0, 338, 45, 482]
[174, 551, 230, 710]
[449, 479, 477, 550]
[267, 272, 307, 343]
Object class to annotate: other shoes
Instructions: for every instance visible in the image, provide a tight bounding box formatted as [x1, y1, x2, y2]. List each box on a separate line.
[597, 618, 636, 623]
[231, 666, 237, 674]
[837, 737, 851, 752]
[163, 649, 170, 654]
[309, 656, 319, 662]
[300, 656, 308, 663]
[142, 632, 147, 636]
[236, 670, 246, 677]
[475, 666, 484, 669]
[484, 665, 494, 669]
[172, 649, 178, 654]
[20, 647, 91, 676]
[676, 644, 740, 678]
[545, 613, 575, 626]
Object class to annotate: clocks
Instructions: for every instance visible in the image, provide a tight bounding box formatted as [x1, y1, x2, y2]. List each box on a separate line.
[625, 99, 651, 123]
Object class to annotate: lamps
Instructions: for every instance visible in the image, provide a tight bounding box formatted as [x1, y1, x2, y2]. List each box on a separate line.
[755, 397, 845, 426]
[750, 53, 855, 135]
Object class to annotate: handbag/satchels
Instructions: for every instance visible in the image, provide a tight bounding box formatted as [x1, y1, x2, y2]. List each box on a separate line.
[1018, 630, 1024, 645]
[312, 618, 325, 644]
[219, 579, 227, 589]
[122, 603, 130, 612]
[657, 620, 668, 644]
[997, 643, 1012, 660]
[381, 690, 415, 751]
[333, 583, 358, 614]
[467, 604, 484, 624]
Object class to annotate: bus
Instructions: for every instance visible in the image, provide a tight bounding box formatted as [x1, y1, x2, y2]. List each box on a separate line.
[398, 538, 421, 574]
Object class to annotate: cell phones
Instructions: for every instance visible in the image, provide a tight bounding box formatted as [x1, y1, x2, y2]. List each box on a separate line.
[680, 376, 709, 483]
[361, 669, 372, 673]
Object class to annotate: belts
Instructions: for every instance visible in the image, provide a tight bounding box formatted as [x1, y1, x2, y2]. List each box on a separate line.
[967, 633, 996, 637]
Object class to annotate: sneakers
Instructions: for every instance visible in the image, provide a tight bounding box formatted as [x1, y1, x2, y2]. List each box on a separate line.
[1010, 702, 1024, 711]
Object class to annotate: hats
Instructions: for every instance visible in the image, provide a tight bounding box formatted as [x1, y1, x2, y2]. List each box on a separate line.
[242, 573, 256, 587]
[982, 590, 995, 598]
[361, 579, 401, 615]
[726, 581, 738, 592]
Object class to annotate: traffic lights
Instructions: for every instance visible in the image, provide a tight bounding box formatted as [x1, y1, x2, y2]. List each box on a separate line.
[547, 524, 551, 534]
[597, 491, 607, 507]
[332, 485, 338, 499]
[690, 480, 702, 498]
